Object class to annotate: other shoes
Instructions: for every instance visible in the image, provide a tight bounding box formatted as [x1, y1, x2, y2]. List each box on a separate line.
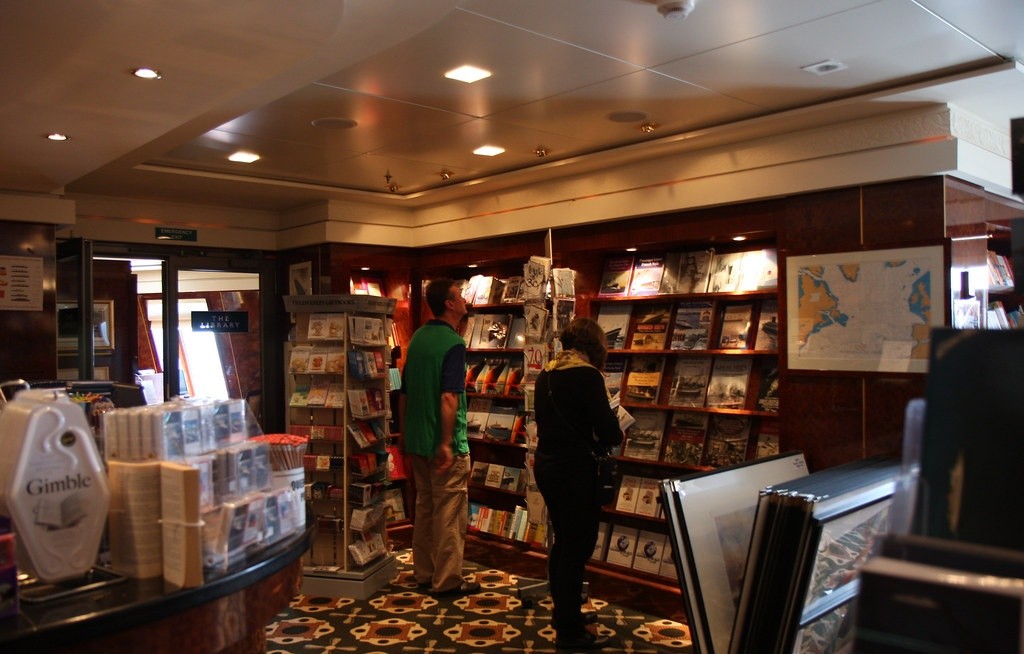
[431, 580, 482, 597]
[582, 611, 599, 625]
[555, 630, 604, 652]
[418, 582, 431, 591]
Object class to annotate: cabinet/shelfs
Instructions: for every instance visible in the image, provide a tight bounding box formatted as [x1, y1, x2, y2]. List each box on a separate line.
[414, 226, 789, 588]
[982, 223, 1024, 329]
[281, 294, 398, 600]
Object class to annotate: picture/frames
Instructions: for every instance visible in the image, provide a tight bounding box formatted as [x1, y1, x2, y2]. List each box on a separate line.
[57, 365, 110, 380]
[654, 450, 904, 653]
[777, 235, 952, 379]
[57, 298, 116, 357]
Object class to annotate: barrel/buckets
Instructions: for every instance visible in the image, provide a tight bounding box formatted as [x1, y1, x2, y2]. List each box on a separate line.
[269, 467, 309, 527]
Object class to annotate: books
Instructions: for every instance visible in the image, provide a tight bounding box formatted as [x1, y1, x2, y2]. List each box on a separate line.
[288, 245, 778, 587]
[987, 250, 1024, 330]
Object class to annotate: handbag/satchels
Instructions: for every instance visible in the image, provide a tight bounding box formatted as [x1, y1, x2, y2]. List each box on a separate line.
[594, 449, 619, 504]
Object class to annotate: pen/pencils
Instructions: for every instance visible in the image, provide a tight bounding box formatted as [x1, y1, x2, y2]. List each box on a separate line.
[247, 434, 309, 472]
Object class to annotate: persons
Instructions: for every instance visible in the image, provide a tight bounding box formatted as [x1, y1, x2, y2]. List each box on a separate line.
[534, 317, 627, 650]
[401, 278, 481, 596]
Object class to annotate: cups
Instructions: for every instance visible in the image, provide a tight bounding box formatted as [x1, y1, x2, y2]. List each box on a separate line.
[140, 374, 163, 404]
